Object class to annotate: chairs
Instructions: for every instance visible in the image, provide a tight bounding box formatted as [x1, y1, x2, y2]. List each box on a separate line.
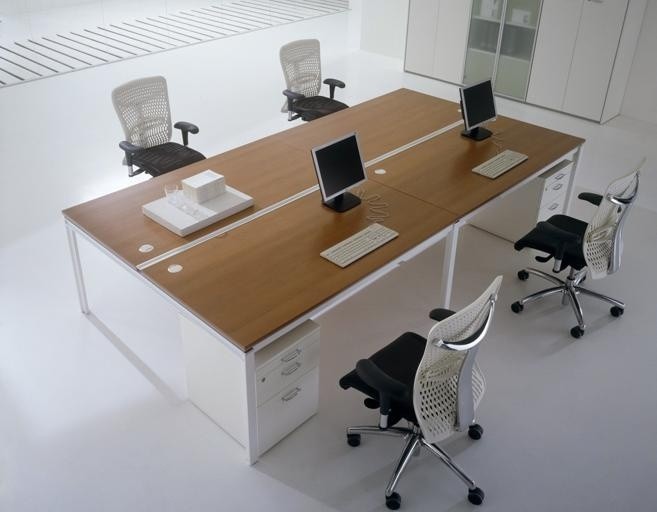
[337, 275, 503, 509]
[276, 38, 351, 124]
[109, 77, 209, 176]
[507, 155, 648, 342]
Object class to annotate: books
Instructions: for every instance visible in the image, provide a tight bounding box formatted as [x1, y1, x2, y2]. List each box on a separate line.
[141, 181, 255, 239]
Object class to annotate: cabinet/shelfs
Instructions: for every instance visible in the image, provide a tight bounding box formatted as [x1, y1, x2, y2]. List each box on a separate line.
[254, 328, 322, 461]
[467, 167, 577, 250]
[400, 0, 650, 123]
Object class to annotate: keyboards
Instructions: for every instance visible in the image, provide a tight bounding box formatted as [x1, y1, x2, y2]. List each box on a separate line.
[472, 148, 529, 181]
[320, 222, 400, 269]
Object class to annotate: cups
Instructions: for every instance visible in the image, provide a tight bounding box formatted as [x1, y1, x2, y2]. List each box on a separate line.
[164, 183, 200, 217]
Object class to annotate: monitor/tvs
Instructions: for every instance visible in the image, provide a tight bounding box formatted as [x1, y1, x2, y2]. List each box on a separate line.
[311, 130, 368, 212]
[460, 80, 497, 141]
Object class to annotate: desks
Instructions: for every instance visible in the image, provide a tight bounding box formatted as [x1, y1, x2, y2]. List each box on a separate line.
[57, 85, 590, 468]
